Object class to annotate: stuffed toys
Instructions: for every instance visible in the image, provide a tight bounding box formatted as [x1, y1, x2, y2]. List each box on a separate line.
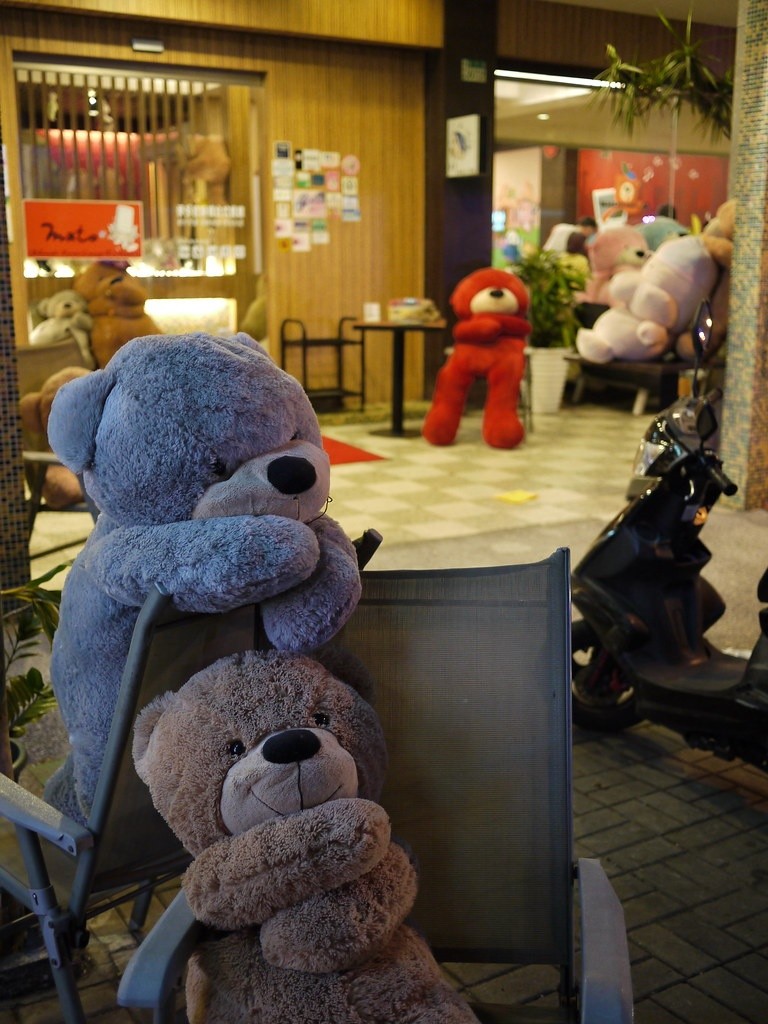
[422, 268, 533, 450]
[42, 332, 361, 829]
[130, 651, 483, 1024]
[17, 367, 93, 509]
[29, 289, 95, 370]
[573, 199, 735, 366]
[76, 260, 161, 371]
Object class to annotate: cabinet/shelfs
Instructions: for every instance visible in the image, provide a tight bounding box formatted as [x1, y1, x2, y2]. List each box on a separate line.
[279, 311, 369, 416]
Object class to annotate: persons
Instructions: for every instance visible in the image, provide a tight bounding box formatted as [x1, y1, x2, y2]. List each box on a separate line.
[551, 217, 597, 255]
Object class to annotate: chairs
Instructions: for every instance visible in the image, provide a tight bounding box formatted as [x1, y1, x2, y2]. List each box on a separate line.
[0, 527, 385, 1024]
[110, 541, 646, 1024]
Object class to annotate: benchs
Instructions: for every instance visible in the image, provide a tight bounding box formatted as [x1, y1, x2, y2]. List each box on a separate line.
[561, 348, 722, 416]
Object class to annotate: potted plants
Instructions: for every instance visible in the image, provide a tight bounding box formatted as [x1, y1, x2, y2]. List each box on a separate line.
[509, 252, 595, 417]
[0, 558, 74, 784]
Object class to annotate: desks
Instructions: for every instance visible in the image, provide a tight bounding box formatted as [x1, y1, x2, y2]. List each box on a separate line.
[351, 314, 450, 439]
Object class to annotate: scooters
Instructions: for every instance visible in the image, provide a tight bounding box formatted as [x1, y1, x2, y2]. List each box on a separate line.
[532, 301, 768, 774]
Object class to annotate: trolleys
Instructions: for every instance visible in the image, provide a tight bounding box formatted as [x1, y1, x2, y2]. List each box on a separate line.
[280, 316, 365, 415]
